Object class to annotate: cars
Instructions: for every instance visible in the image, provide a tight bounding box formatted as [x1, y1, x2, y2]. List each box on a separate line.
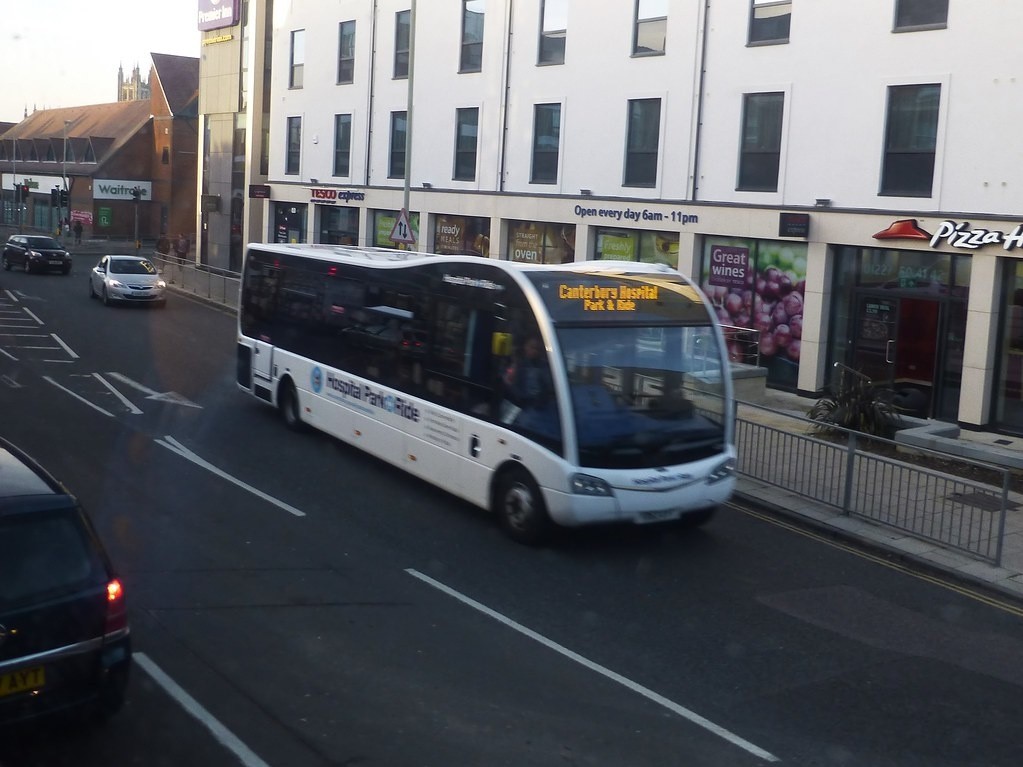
[91, 254, 168, 307]
[0, 434, 135, 758]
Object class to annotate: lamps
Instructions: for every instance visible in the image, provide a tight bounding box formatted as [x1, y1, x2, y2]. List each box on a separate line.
[815, 199, 830, 207]
[580, 190, 590, 195]
[310, 179, 316, 183]
[422, 183, 431, 188]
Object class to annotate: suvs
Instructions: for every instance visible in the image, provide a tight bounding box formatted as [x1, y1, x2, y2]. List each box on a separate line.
[3, 234, 72, 276]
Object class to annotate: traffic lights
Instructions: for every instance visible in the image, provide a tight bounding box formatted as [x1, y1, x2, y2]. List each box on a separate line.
[60, 189, 67, 208]
[23, 186, 29, 197]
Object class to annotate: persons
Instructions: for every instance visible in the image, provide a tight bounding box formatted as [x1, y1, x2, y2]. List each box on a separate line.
[156, 232, 170, 260]
[176, 233, 188, 272]
[73, 221, 83, 244]
[500, 336, 556, 412]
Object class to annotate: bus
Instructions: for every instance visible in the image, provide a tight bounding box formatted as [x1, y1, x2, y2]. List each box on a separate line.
[235, 242, 741, 546]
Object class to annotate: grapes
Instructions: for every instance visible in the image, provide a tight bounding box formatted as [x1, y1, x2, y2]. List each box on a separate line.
[703, 240, 806, 363]
